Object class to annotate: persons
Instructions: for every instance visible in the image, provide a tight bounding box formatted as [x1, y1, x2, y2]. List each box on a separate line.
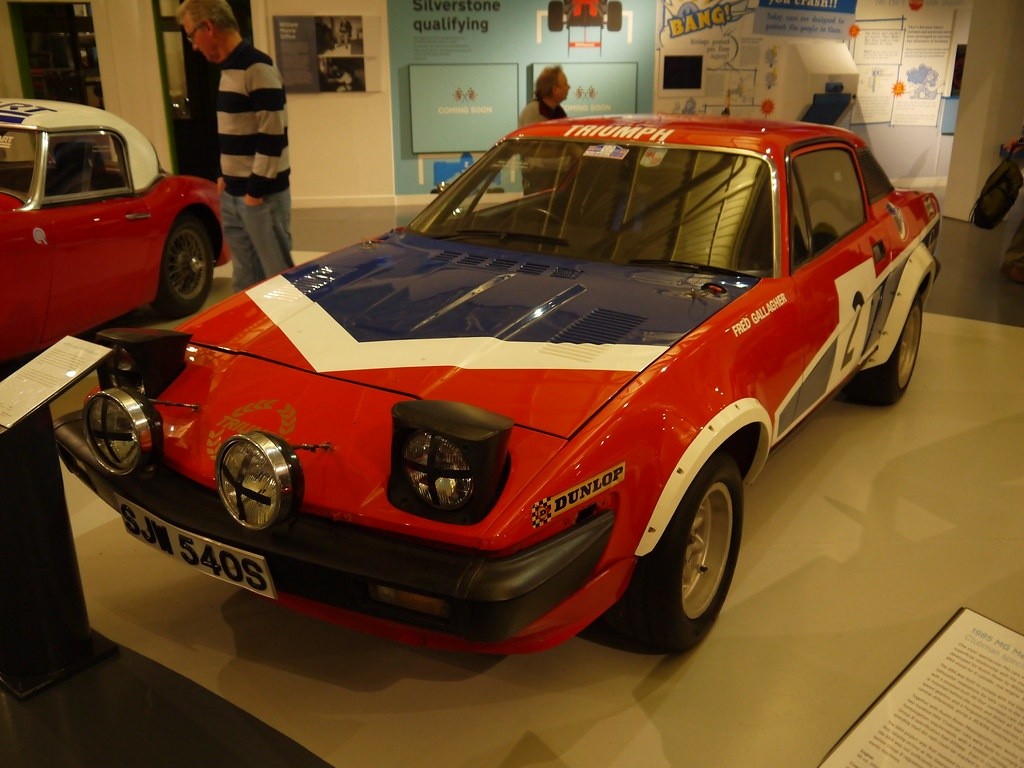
[175, 0, 296, 293]
[519, 66, 571, 195]
[337, 17, 351, 49]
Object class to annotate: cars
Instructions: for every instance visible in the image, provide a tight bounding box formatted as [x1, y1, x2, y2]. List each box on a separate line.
[51, 115, 938, 658]
[0, 98, 234, 368]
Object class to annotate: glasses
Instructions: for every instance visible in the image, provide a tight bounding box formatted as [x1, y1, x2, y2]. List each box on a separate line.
[186, 22, 207, 41]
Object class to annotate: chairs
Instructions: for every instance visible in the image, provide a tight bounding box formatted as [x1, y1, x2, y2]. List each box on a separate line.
[43, 139, 93, 196]
